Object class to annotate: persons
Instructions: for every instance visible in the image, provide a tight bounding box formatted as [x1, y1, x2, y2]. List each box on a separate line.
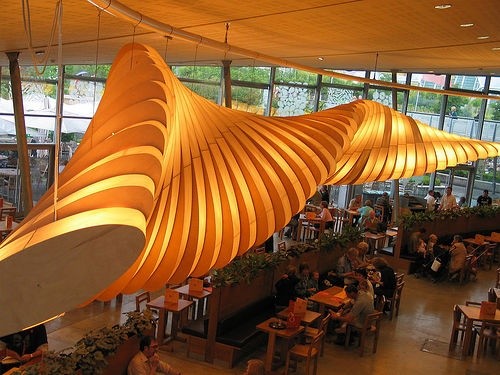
[273, 183, 500, 357]
[127, 336, 182, 374]
[22, 324, 50, 361]
[0, 341, 23, 375]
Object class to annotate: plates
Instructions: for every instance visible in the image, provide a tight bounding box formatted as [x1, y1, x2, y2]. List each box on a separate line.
[269, 322, 286, 330]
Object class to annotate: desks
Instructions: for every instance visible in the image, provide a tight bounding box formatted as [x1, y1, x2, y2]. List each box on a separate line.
[40, 166, 66, 174]
[173, 284, 211, 322]
[256, 317, 304, 375]
[461, 238, 489, 247]
[344, 209, 361, 226]
[365, 233, 386, 251]
[146, 295, 196, 352]
[297, 214, 335, 240]
[0, 168, 33, 202]
[307, 285, 349, 329]
[387, 231, 397, 247]
[277, 308, 322, 346]
[450, 306, 500, 357]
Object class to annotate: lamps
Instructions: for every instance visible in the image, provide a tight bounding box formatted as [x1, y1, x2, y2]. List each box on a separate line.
[0, 43, 500, 338]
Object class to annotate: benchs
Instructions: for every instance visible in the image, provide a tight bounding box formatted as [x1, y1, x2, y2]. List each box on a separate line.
[182, 237, 364, 368]
[376, 206, 500, 275]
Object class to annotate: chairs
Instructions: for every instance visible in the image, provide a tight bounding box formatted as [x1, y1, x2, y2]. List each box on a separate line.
[449, 305, 476, 353]
[0, 207, 16, 222]
[136, 291, 159, 324]
[471, 320, 500, 359]
[461, 301, 481, 341]
[286, 244, 500, 375]
[37, 156, 49, 190]
[279, 199, 394, 230]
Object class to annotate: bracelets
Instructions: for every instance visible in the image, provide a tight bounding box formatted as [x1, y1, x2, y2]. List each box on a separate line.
[30, 354, 33, 359]
